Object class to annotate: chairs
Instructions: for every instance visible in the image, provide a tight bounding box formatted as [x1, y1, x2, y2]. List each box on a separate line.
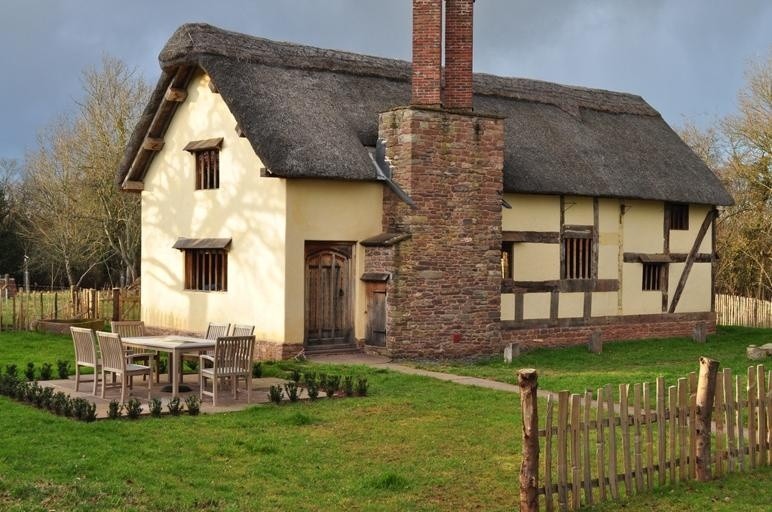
[70, 321, 255, 407]
